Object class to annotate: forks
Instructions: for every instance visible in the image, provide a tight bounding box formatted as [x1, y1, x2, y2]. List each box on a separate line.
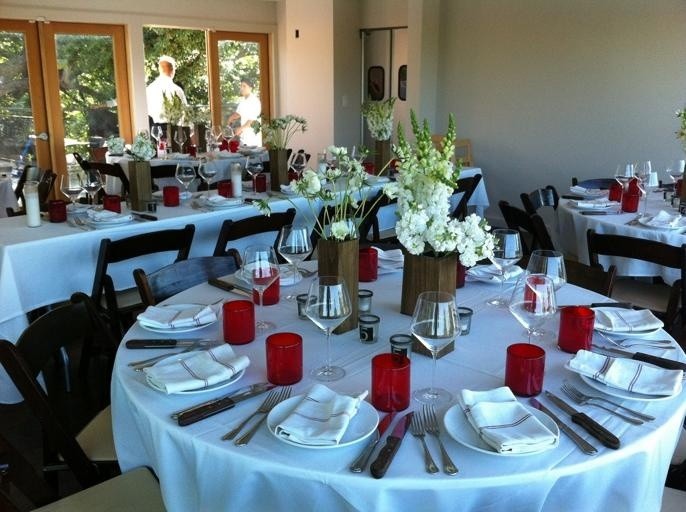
[406, 411, 439, 475]
[606, 336, 677, 350]
[598, 332, 671, 345]
[421, 404, 459, 476]
[221, 390, 281, 441]
[559, 385, 644, 426]
[130, 343, 213, 372]
[235, 386, 293, 447]
[565, 384, 657, 422]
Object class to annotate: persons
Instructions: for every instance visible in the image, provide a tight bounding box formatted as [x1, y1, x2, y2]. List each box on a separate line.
[85, 96, 117, 183]
[145, 54, 188, 142]
[225, 78, 263, 149]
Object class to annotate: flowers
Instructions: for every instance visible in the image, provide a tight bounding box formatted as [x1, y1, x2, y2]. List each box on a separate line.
[246, 112, 311, 149]
[157, 91, 193, 124]
[361, 95, 400, 140]
[382, 108, 503, 273]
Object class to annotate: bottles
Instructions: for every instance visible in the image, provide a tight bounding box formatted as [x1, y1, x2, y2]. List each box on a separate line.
[25, 181, 41, 226]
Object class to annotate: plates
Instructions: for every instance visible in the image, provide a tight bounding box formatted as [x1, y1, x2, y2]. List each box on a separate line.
[270, 391, 381, 450]
[444, 396, 561, 458]
[578, 359, 683, 402]
[146, 353, 247, 394]
[640, 215, 686, 230]
[242, 259, 303, 287]
[592, 306, 662, 337]
[139, 302, 217, 333]
[574, 201, 615, 210]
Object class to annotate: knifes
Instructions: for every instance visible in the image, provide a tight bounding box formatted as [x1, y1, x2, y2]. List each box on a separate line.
[563, 195, 606, 201]
[591, 344, 686, 371]
[556, 302, 635, 310]
[582, 211, 626, 216]
[544, 391, 621, 449]
[171, 383, 268, 419]
[208, 275, 256, 300]
[351, 411, 397, 472]
[528, 397, 599, 455]
[179, 385, 277, 426]
[370, 411, 414, 479]
[127, 338, 221, 348]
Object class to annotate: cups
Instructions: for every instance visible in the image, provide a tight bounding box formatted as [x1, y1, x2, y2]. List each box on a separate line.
[358, 248, 378, 283]
[389, 334, 414, 358]
[609, 174, 641, 211]
[357, 314, 381, 344]
[525, 276, 546, 311]
[370, 353, 412, 412]
[264, 332, 305, 387]
[505, 342, 547, 398]
[296, 292, 318, 320]
[357, 289, 374, 313]
[558, 305, 594, 354]
[47, 141, 302, 224]
[222, 300, 256, 345]
[251, 267, 280, 306]
[458, 306, 473, 335]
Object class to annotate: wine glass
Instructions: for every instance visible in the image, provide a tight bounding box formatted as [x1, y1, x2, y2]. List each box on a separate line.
[306, 275, 353, 383]
[241, 248, 282, 330]
[615, 159, 686, 219]
[58, 123, 369, 218]
[525, 249, 567, 308]
[279, 224, 315, 303]
[410, 291, 462, 405]
[510, 275, 558, 344]
[486, 229, 523, 308]
